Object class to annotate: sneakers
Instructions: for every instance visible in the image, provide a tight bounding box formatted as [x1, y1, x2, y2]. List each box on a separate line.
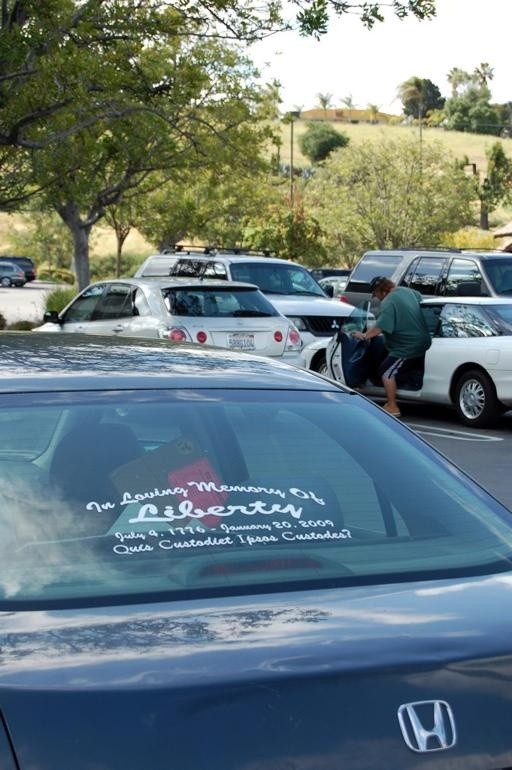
[381, 402, 403, 419]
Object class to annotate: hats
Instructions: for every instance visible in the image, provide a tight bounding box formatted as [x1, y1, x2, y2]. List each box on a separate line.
[369, 275, 386, 299]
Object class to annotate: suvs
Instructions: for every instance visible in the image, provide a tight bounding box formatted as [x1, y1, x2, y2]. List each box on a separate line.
[340, 252, 512, 297]
[135, 245, 377, 344]
[0, 256, 40, 283]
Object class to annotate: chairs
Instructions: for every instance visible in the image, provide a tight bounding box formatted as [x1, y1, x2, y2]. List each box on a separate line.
[48, 424, 345, 540]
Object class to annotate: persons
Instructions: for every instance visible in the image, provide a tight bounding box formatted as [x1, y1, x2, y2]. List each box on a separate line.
[0, 469, 138, 588]
[351, 276, 431, 417]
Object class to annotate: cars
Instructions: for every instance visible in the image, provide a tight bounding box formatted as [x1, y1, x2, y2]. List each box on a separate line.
[30, 279, 303, 367]
[302, 296, 511, 429]
[0, 262, 27, 287]
[311, 266, 353, 279]
[308, 275, 351, 298]
[3, 328, 509, 770]
